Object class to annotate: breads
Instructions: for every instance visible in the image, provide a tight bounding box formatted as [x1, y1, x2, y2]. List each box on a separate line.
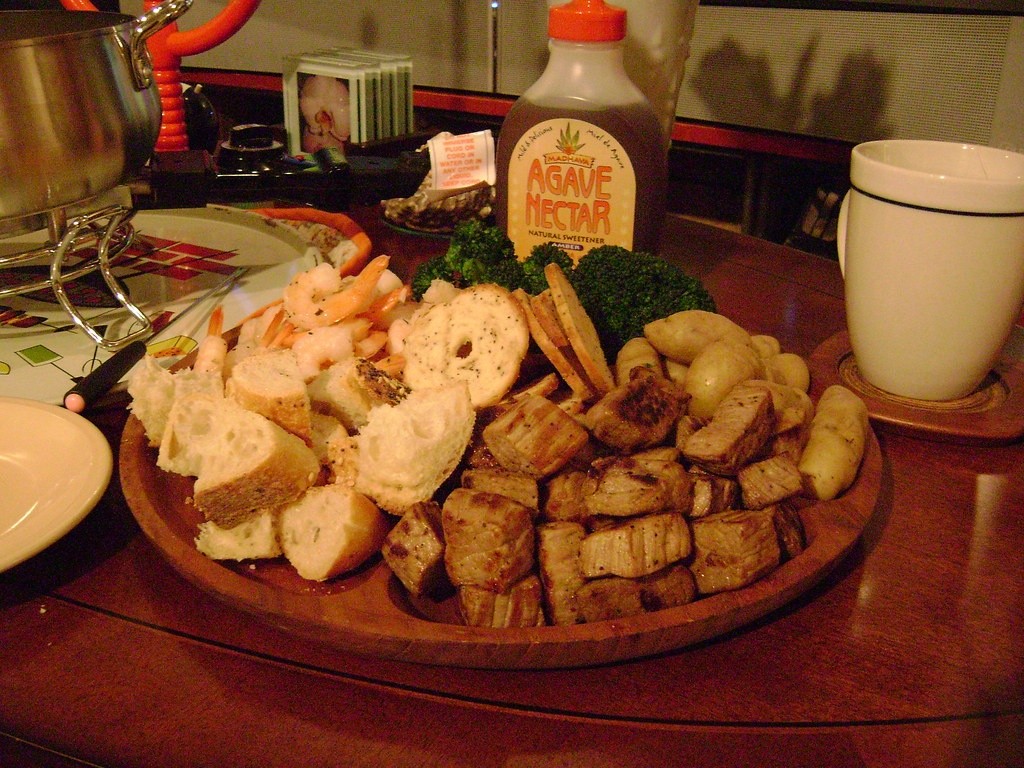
[125, 284, 530, 584]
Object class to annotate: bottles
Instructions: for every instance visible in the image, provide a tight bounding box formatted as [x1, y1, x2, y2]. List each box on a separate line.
[497, 0, 667, 272]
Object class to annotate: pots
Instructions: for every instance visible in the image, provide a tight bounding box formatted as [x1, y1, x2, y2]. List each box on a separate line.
[0, 0, 192, 220]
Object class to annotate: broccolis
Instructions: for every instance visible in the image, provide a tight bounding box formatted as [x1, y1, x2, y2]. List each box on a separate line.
[411, 220, 715, 366]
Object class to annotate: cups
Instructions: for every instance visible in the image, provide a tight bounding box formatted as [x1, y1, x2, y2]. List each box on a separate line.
[835, 137, 1024, 400]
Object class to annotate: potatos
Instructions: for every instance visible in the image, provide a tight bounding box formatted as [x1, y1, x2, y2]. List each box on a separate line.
[613, 310, 869, 502]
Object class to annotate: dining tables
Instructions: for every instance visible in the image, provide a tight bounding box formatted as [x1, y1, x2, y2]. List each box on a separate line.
[0, 214, 1024, 768]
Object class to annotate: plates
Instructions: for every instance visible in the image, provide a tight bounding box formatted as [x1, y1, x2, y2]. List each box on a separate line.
[379, 214, 454, 240]
[119, 392, 882, 670]
[0, 397, 113, 572]
[811, 330, 1024, 439]
